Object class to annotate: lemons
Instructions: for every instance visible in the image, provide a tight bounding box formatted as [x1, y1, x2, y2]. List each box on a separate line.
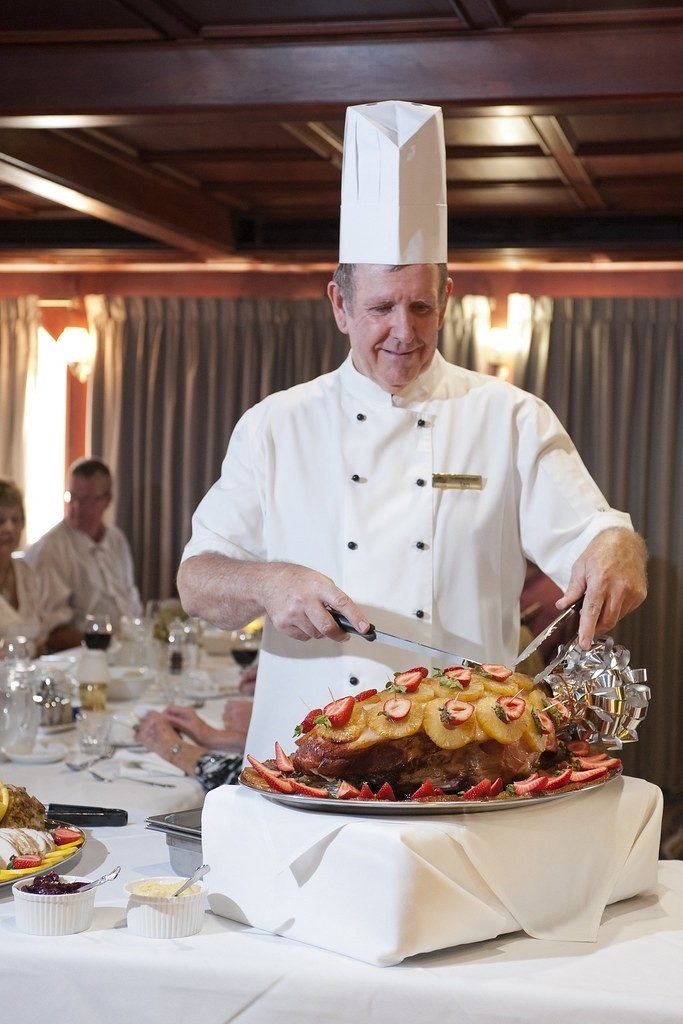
[0, 782, 9, 822]
[0, 838, 83, 882]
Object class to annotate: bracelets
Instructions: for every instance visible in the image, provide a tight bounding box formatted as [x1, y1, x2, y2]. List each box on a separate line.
[165, 740, 186, 764]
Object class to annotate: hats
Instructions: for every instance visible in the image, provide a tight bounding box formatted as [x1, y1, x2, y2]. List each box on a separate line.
[338, 100, 448, 263]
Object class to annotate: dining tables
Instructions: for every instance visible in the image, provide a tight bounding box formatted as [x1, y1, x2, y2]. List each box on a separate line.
[0, 610, 683, 1024]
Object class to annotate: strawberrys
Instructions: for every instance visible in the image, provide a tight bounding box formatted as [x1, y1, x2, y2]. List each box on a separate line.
[54, 830, 82, 844]
[247, 664, 619, 801]
[14, 855, 42, 868]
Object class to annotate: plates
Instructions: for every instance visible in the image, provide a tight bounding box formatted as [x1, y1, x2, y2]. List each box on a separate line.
[240, 751, 625, 819]
[0, 819, 88, 897]
[1, 737, 69, 763]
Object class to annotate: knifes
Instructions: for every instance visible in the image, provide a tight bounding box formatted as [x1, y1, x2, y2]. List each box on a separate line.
[322, 612, 485, 671]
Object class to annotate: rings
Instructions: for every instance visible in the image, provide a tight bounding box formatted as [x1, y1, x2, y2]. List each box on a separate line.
[134, 723, 139, 731]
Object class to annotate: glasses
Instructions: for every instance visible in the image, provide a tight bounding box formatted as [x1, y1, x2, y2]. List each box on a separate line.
[64, 490, 110, 504]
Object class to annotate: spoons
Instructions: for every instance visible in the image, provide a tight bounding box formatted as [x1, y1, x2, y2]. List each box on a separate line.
[90, 769, 175, 789]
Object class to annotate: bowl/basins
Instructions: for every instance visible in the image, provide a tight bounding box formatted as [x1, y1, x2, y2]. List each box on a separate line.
[107, 666, 156, 700]
[122, 874, 209, 939]
[12, 876, 95, 937]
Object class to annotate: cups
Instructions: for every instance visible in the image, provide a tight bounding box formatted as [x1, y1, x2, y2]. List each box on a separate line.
[75, 711, 118, 759]
[80, 611, 115, 653]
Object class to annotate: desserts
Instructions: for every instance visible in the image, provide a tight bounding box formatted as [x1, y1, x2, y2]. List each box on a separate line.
[18, 873, 89, 894]
[132, 881, 200, 897]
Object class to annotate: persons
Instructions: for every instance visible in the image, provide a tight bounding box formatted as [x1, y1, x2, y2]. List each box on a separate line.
[0, 478, 78, 671]
[24, 459, 146, 651]
[222, 661, 261, 737]
[130, 691, 251, 798]
[175, 236, 647, 793]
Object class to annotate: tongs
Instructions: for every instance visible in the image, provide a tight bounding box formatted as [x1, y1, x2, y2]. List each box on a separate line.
[41, 803, 128, 828]
[511, 593, 606, 683]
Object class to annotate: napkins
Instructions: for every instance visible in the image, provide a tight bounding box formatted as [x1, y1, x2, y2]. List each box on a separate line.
[121, 750, 186, 777]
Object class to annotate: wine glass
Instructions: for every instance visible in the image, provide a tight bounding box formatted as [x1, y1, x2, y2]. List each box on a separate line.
[230, 626, 260, 681]
